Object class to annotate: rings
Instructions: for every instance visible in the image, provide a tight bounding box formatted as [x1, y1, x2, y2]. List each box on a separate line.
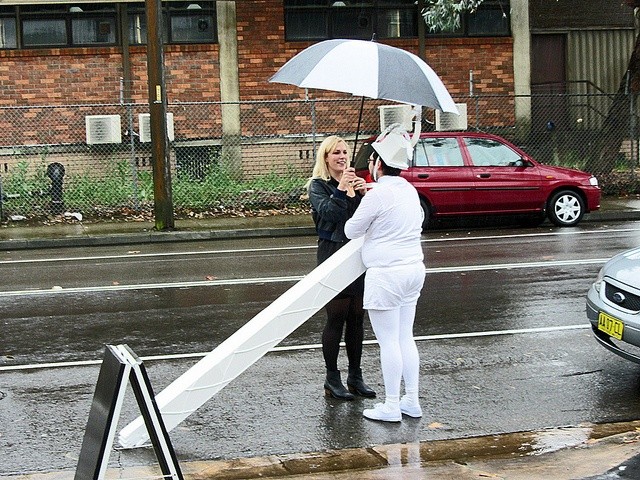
[358, 184, 362, 187]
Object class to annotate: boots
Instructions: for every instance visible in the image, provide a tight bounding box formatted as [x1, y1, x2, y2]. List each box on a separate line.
[347, 366, 376, 397]
[324, 370, 355, 400]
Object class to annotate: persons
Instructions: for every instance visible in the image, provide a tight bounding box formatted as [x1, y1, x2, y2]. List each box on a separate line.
[344, 122, 425, 423]
[306, 135, 376, 400]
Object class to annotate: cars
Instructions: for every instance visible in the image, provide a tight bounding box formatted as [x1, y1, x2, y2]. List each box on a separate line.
[353, 132, 602, 227]
[586, 245, 640, 364]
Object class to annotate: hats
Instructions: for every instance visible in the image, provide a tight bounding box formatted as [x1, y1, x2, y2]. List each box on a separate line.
[371, 123, 414, 171]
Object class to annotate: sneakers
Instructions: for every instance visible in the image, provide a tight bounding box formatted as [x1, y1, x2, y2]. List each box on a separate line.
[399, 399, 422, 418]
[363, 405, 402, 422]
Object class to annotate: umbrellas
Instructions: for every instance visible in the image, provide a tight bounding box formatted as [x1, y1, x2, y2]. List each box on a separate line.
[268, 32, 460, 168]
